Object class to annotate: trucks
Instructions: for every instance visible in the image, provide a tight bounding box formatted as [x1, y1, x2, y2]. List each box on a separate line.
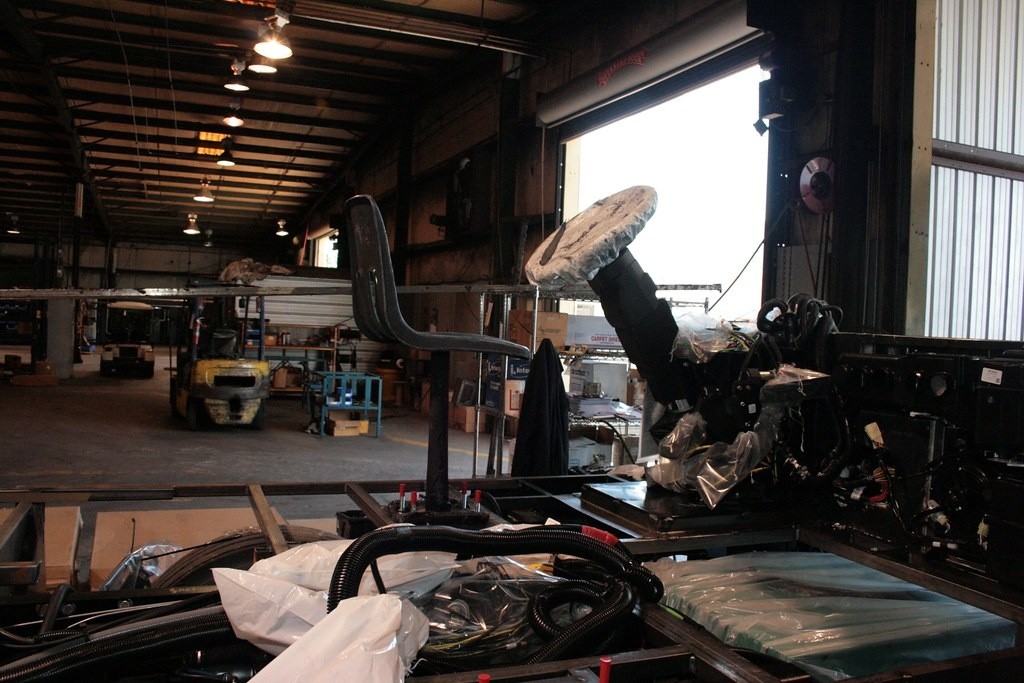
[99, 303, 155, 379]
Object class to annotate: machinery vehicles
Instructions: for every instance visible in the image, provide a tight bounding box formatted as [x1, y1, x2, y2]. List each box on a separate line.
[164, 291, 276, 431]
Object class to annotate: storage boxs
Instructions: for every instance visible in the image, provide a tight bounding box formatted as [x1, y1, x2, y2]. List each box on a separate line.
[88, 508, 339, 592]
[423, 383, 487, 433]
[246, 329, 260, 345]
[329, 417, 369, 437]
[569, 425, 640, 467]
[265, 332, 277, 346]
[569, 362, 646, 407]
[508, 309, 623, 354]
[1, 508, 82, 592]
[273, 367, 302, 388]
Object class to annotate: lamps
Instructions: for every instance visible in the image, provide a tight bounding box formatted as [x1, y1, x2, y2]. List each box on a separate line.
[183, 214, 200, 234]
[223, 50, 250, 91]
[6, 215, 20, 234]
[223, 97, 244, 127]
[247, 54, 277, 74]
[202, 229, 213, 247]
[216, 138, 236, 167]
[276, 219, 289, 236]
[193, 179, 214, 202]
[254, 0, 298, 59]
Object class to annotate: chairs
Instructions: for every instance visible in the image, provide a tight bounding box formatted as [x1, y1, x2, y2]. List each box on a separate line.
[344, 196, 532, 512]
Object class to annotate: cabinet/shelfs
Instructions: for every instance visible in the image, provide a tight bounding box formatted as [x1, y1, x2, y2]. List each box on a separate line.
[473, 292, 642, 479]
[237, 322, 338, 413]
